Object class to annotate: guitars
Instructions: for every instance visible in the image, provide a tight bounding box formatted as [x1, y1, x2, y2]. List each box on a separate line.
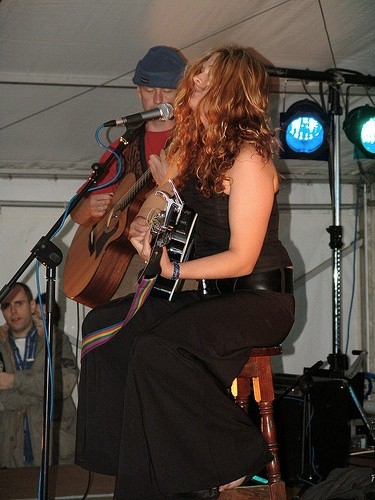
[63, 145, 184, 310]
[137, 197, 198, 304]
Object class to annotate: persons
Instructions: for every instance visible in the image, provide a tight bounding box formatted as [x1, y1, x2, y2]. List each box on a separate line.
[65, 45, 198, 305]
[32, 292, 62, 325]
[0, 282, 80, 468]
[71, 48, 295, 499]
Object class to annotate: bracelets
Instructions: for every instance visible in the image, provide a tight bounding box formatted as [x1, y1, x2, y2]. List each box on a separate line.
[171, 261, 180, 281]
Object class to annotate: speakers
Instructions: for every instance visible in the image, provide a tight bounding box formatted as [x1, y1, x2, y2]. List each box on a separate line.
[271, 372, 352, 491]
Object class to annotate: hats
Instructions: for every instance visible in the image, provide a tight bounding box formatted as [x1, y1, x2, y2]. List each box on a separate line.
[132, 46, 186, 88]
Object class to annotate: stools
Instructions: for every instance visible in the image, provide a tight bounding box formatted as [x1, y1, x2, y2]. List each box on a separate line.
[215, 346, 288, 500]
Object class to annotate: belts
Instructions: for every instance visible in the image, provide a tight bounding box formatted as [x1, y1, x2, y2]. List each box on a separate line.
[198, 267, 294, 296]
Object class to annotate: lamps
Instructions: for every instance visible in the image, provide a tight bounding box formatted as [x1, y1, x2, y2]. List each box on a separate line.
[343, 85, 375, 161]
[279, 100, 333, 163]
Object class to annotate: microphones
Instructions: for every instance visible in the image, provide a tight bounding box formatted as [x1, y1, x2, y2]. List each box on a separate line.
[102, 103, 173, 128]
